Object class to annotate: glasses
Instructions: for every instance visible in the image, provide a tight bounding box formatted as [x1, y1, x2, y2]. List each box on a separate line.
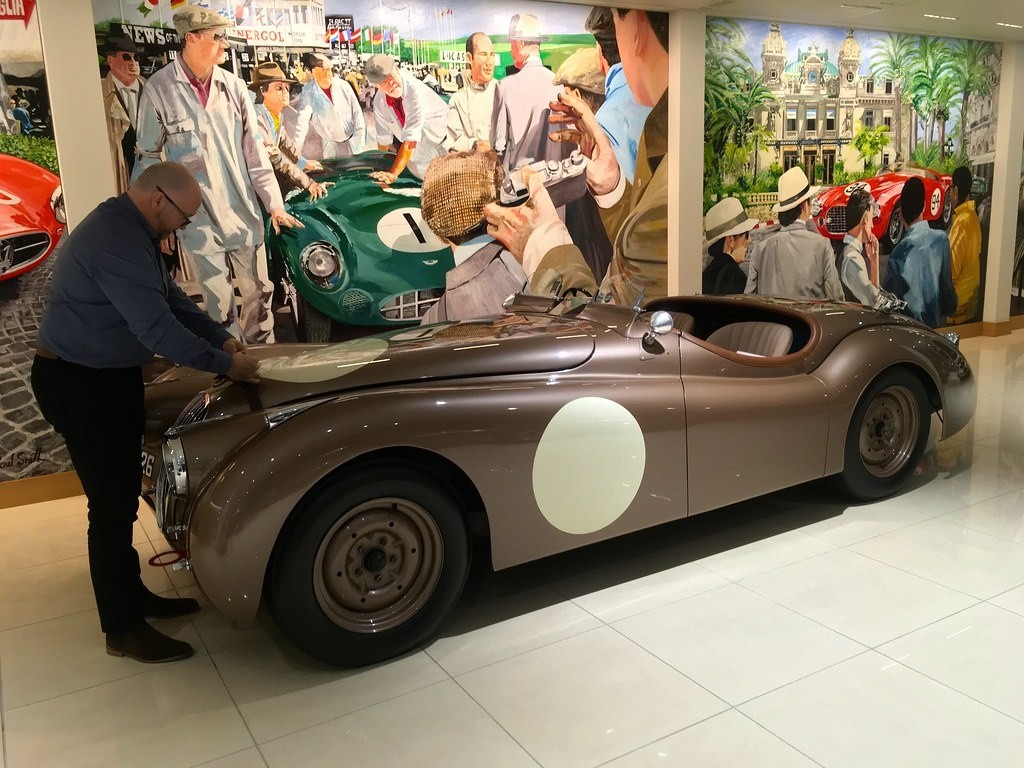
[156, 185, 192, 227]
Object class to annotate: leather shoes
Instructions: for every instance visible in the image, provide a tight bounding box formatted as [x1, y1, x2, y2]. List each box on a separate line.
[104, 625, 192, 662]
[142, 589, 200, 619]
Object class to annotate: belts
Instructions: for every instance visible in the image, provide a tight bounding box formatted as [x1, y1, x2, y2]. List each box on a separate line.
[36, 348, 58, 359]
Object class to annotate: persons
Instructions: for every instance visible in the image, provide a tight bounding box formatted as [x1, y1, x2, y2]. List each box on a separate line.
[28, 160, 261, 665]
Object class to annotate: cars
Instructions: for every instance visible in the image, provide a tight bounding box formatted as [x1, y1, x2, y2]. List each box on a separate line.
[141, 274, 978, 668]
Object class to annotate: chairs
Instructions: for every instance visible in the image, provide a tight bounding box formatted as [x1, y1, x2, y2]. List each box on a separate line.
[702, 320, 793, 357]
[639, 309, 692, 334]
